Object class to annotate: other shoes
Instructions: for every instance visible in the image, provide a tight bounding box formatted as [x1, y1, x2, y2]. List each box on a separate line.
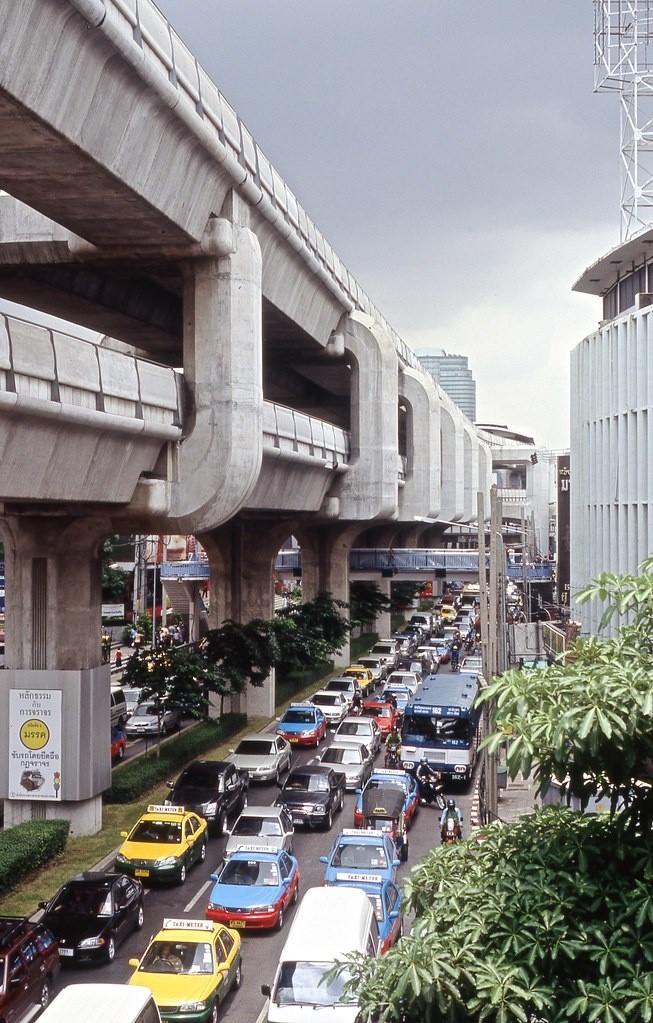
[383, 764, 386, 767]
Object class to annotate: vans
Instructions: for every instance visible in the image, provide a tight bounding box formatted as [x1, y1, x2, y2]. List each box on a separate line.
[111, 687, 128, 730]
[33, 984, 162, 1023]
[262, 886, 384, 1023]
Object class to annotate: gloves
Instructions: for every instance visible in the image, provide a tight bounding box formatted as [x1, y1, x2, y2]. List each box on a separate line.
[422, 781, 427, 785]
[434, 774, 439, 779]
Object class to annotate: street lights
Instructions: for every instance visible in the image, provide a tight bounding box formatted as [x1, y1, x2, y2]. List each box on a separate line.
[413, 515, 507, 673]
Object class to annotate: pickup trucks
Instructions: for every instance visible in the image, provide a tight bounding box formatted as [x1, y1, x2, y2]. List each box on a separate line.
[164, 761, 250, 837]
[274, 765, 347, 831]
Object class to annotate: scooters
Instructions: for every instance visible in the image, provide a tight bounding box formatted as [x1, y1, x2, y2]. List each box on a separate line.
[385, 742, 400, 768]
[438, 816, 463, 845]
[420, 773, 446, 811]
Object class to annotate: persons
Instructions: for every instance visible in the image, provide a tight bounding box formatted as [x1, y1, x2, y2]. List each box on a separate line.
[158, 621, 184, 649]
[440, 800, 463, 832]
[102, 631, 111, 661]
[450, 646, 462, 667]
[153, 944, 183, 972]
[202, 583, 207, 598]
[385, 691, 397, 709]
[532, 554, 549, 569]
[113, 647, 122, 673]
[127, 624, 145, 653]
[142, 822, 159, 840]
[352, 690, 363, 715]
[55, 889, 88, 915]
[416, 757, 438, 804]
[382, 725, 402, 768]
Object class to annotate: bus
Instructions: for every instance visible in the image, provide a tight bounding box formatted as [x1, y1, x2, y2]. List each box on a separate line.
[102, 562, 173, 621]
[401, 674, 482, 794]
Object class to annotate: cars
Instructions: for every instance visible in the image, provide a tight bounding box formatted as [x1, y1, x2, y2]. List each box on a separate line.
[123, 676, 197, 739]
[126, 918, 242, 1023]
[223, 574, 482, 791]
[115, 805, 208, 888]
[319, 770, 420, 955]
[223, 805, 295, 865]
[206, 845, 300, 932]
[111, 726, 126, 760]
[37, 873, 144, 966]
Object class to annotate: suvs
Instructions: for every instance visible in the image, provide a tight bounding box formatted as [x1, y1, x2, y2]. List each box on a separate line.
[0, 916, 61, 1023]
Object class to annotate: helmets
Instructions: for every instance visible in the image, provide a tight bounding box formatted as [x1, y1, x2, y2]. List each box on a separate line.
[420, 757, 428, 767]
[447, 800, 455, 810]
[355, 691, 359, 697]
[453, 645, 458, 652]
[392, 726, 397, 734]
